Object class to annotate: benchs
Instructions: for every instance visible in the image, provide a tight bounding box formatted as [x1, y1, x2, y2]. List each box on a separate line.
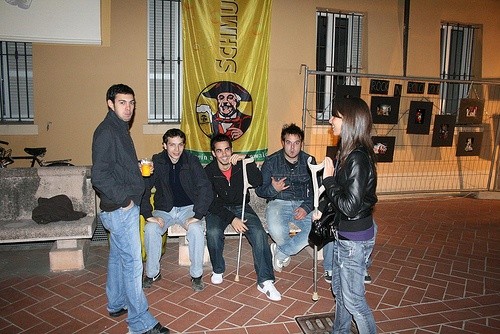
[168, 188, 323, 267]
[0, 166, 97, 272]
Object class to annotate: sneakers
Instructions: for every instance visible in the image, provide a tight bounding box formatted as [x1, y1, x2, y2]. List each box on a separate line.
[143, 272, 161, 288]
[109, 308, 128, 317]
[190, 274, 204, 292]
[142, 322, 170, 334]
[210, 270, 224, 285]
[256, 279, 282, 302]
[364, 271, 372, 284]
[270, 242, 291, 273]
[323, 270, 333, 283]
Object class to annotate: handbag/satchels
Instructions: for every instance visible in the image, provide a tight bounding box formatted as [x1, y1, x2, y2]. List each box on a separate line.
[308, 209, 337, 251]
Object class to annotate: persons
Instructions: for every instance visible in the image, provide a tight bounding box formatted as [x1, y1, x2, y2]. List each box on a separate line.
[204, 133, 281, 301]
[322, 139, 377, 284]
[255, 123, 321, 273]
[140, 128, 214, 292]
[312, 96, 378, 334]
[91, 84, 170, 334]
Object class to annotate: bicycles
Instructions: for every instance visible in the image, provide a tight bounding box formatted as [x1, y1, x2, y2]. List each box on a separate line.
[0, 140, 76, 166]
